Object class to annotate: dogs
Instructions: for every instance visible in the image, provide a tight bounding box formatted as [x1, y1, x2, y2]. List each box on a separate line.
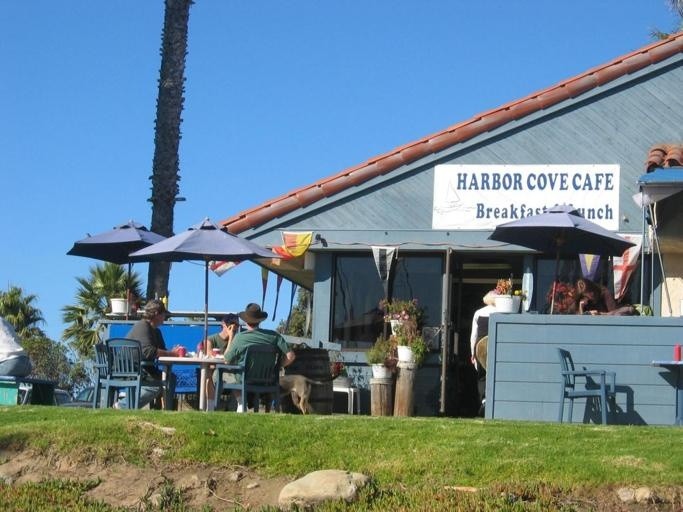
[279, 372, 341, 416]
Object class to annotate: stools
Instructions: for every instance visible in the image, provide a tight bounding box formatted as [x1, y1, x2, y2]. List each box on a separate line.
[332, 388, 360, 415]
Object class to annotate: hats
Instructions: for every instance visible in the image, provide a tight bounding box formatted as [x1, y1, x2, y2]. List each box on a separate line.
[239, 303, 267, 325]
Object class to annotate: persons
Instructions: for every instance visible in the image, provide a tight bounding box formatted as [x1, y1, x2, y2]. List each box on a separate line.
[112, 299, 188, 411]
[574, 278, 616, 314]
[0, 317, 32, 377]
[470, 290, 495, 416]
[224, 303, 296, 414]
[202, 313, 245, 414]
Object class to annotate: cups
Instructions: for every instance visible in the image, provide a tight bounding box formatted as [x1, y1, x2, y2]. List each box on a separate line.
[178, 347, 186, 357]
[674, 345, 681, 361]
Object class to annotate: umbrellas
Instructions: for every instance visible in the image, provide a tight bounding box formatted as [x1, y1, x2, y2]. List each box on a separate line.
[127, 217, 284, 356]
[487, 204, 637, 314]
[65, 220, 183, 314]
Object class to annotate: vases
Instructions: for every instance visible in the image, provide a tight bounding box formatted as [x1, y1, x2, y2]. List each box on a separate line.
[390, 320, 404, 337]
[398, 345, 414, 362]
[494, 295, 522, 313]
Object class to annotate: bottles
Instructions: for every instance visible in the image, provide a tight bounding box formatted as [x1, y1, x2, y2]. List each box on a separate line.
[199, 350, 203, 359]
[213, 349, 221, 357]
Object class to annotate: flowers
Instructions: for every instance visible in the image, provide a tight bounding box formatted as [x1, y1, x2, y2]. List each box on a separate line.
[495, 278, 513, 295]
[397, 332, 428, 362]
[378, 297, 422, 321]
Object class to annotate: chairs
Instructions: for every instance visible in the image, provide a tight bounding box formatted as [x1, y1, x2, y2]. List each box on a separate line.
[557, 348, 616, 425]
[92, 338, 282, 414]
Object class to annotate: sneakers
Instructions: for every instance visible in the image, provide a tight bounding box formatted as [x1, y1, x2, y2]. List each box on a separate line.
[237, 403, 248, 413]
[479, 403, 485, 415]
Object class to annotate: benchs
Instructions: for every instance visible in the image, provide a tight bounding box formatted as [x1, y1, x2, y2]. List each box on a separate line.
[0, 375, 59, 404]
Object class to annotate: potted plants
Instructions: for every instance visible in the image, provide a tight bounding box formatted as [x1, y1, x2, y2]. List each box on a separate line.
[369, 340, 393, 378]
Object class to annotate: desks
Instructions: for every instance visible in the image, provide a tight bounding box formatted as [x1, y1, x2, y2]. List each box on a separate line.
[653, 361, 683, 426]
